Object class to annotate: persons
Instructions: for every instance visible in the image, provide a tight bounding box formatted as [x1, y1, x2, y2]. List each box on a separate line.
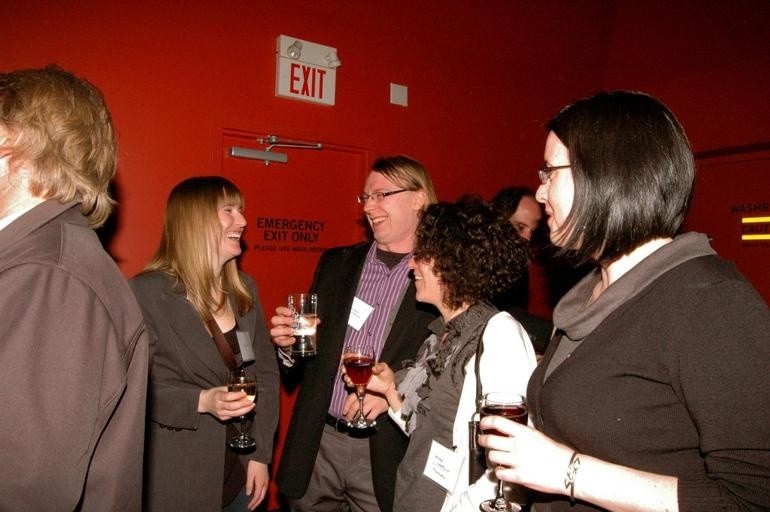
[274, 154, 441, 512]
[2, 62, 151, 512]
[474, 88, 770, 512]
[491, 183, 553, 355]
[339, 191, 539, 512]
[127, 175, 284, 511]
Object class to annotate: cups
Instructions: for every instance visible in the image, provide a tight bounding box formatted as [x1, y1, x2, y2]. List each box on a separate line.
[287, 293, 318, 357]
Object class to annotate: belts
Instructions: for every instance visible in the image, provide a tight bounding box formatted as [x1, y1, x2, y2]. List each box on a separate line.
[325, 414, 377, 439]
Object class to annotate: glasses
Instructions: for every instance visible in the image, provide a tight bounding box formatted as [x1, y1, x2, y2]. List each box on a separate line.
[538, 164, 572, 185]
[355, 188, 405, 206]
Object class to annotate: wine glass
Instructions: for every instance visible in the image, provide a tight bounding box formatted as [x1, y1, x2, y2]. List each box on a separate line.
[479, 391, 529, 512]
[343, 345, 375, 429]
[226, 369, 258, 448]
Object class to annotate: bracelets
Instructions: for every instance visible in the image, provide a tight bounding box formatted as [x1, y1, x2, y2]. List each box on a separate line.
[564, 449, 581, 506]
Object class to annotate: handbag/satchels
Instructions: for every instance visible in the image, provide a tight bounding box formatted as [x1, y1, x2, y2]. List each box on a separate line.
[469, 421, 489, 486]
